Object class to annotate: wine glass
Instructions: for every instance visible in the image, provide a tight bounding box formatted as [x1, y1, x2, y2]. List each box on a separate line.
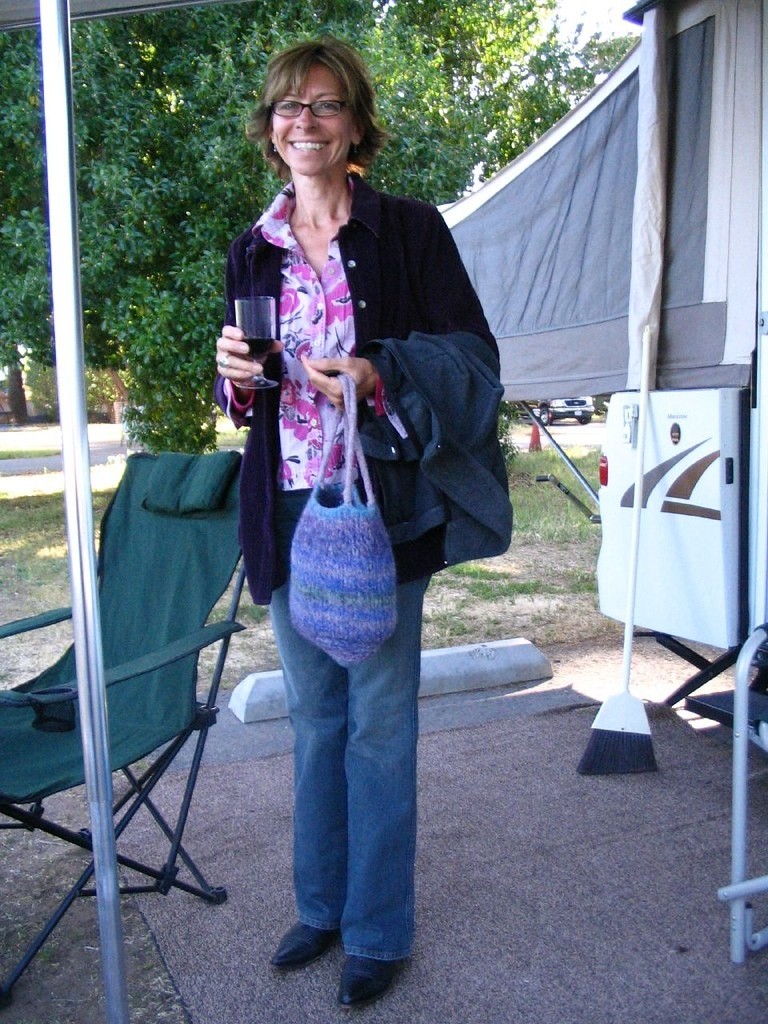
[230, 295, 280, 391]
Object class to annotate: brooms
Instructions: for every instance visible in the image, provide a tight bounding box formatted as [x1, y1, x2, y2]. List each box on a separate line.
[575, 323, 660, 778]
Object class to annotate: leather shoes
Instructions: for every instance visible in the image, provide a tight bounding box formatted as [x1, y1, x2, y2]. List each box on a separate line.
[339, 951, 394, 1008]
[273, 919, 341, 968]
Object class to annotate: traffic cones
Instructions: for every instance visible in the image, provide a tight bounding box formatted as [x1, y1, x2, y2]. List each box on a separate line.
[527, 422, 543, 452]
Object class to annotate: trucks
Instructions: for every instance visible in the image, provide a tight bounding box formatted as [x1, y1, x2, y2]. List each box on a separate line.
[0, 0, 767, 1024]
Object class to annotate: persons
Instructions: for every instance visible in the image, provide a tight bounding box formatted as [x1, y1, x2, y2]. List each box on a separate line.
[212, 39, 514, 1011]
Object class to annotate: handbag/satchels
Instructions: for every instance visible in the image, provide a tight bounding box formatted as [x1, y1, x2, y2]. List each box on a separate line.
[290, 373, 398, 670]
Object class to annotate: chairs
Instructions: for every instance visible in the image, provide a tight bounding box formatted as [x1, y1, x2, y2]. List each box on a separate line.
[1, 451, 249, 1009]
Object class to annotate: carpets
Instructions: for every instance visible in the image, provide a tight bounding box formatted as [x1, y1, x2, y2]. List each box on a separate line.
[112, 700, 768, 1024]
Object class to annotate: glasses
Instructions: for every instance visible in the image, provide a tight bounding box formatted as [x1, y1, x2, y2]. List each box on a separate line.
[272, 97, 353, 117]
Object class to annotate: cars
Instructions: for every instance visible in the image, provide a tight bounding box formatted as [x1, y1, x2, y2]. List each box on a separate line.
[515, 395, 594, 426]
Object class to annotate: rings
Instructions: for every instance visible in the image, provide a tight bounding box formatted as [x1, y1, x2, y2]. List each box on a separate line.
[219, 352, 230, 367]
